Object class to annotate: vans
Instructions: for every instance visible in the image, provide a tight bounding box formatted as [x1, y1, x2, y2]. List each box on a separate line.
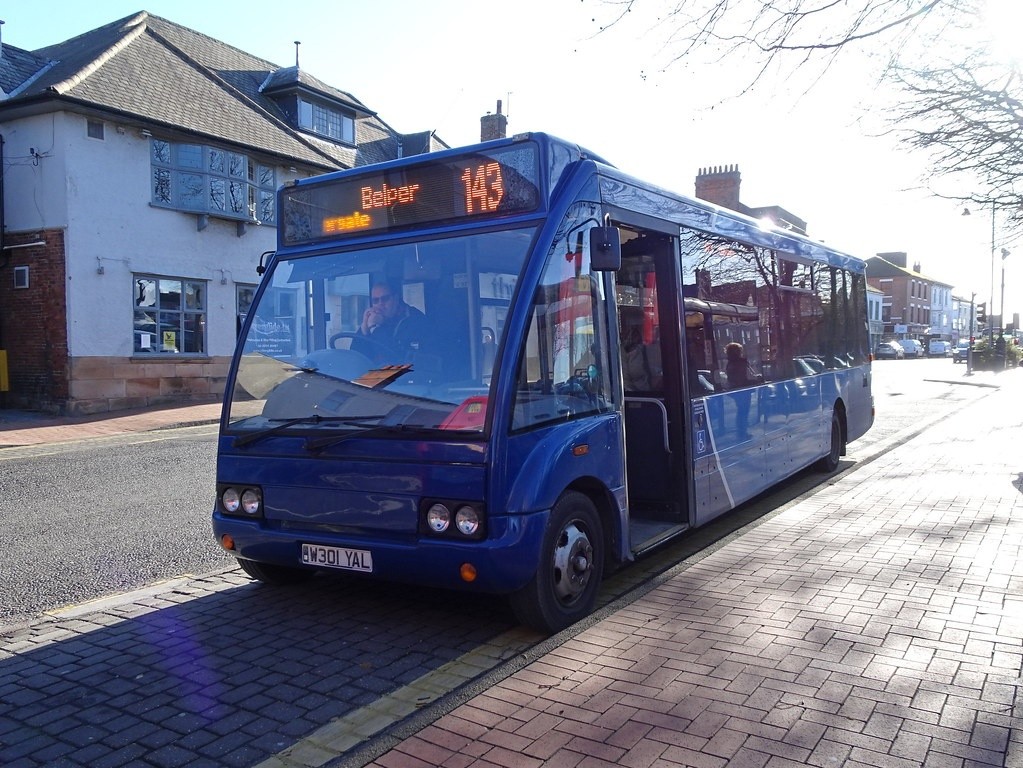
[928, 338, 952, 358]
[899, 340, 924, 358]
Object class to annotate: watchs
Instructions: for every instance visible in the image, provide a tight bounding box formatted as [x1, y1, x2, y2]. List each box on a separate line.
[365, 323, 383, 332]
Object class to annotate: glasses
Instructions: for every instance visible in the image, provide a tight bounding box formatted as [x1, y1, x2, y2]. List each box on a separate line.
[372, 293, 392, 304]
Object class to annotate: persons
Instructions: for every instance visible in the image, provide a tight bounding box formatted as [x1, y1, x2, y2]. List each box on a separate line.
[482, 336, 497, 364]
[350, 281, 426, 368]
[725, 344, 757, 439]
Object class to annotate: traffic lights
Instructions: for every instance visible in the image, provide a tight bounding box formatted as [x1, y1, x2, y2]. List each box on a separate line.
[976, 302, 987, 323]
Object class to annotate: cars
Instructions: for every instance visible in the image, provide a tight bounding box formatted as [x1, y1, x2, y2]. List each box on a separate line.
[953, 340, 970, 363]
[876, 341, 905, 360]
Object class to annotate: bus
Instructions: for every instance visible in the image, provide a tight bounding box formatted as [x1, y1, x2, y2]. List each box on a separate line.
[213, 130, 876, 634]
